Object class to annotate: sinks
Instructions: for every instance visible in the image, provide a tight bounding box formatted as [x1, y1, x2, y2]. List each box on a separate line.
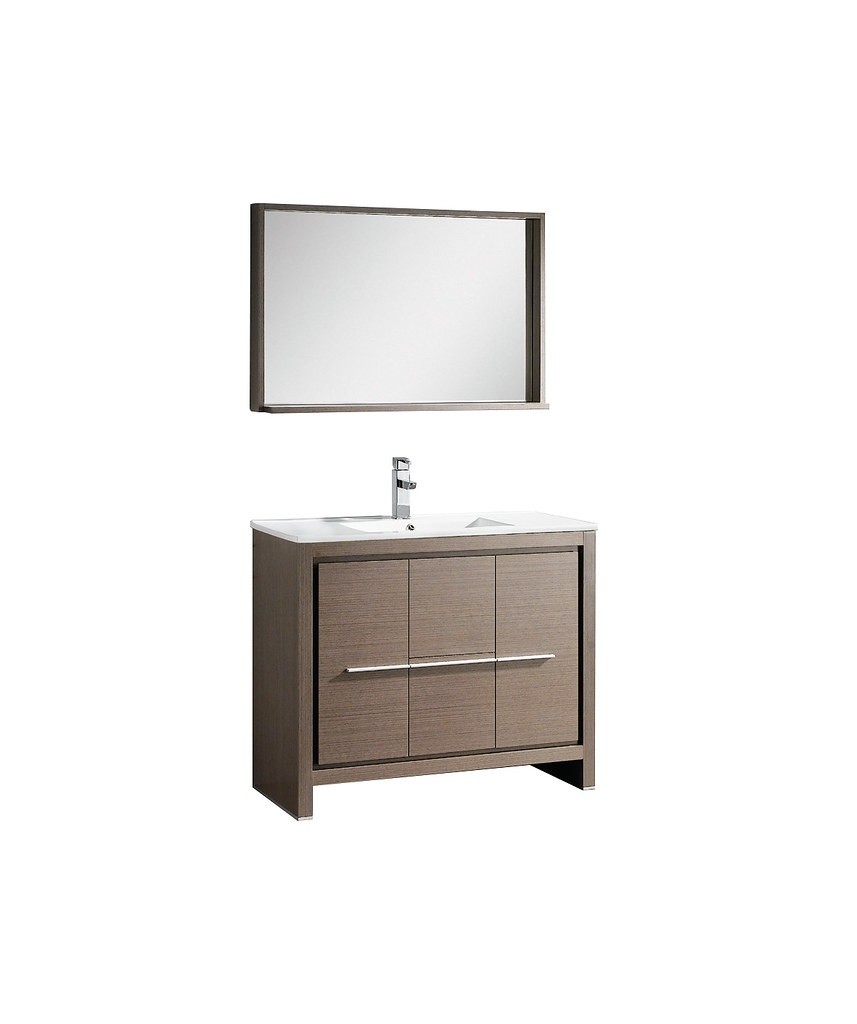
[336, 515, 517, 534]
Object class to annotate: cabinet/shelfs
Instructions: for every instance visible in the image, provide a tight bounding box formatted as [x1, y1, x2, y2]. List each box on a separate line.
[240, 526, 605, 822]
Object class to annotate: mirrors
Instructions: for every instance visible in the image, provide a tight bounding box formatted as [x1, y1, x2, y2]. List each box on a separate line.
[244, 199, 549, 415]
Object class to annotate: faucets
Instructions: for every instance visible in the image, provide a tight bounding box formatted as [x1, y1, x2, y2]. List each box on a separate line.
[390, 456, 417, 519]
